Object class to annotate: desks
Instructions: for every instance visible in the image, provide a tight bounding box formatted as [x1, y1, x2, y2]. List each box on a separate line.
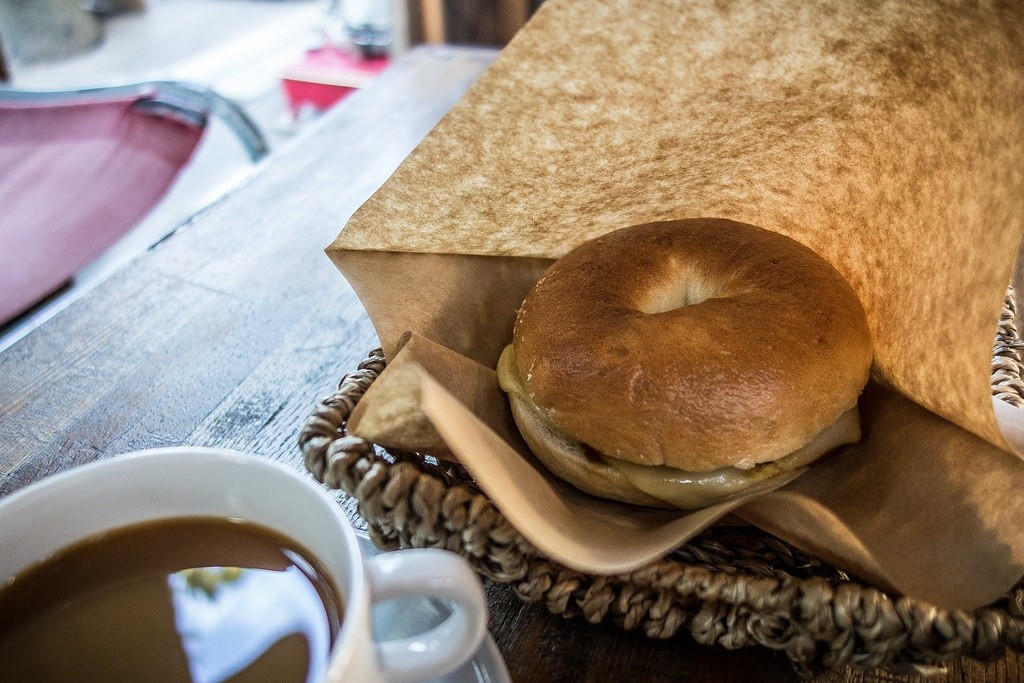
[0, 45, 1024, 683]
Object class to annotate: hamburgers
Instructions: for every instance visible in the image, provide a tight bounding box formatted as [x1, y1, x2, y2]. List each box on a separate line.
[497, 217, 876, 511]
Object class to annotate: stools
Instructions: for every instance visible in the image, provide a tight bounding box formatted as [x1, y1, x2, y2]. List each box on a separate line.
[2, 83, 267, 327]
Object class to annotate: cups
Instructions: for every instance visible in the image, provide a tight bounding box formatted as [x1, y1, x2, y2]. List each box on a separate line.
[0, 445, 488, 682]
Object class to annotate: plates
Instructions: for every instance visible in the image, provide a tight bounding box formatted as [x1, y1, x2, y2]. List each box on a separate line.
[358, 530, 514, 682]
